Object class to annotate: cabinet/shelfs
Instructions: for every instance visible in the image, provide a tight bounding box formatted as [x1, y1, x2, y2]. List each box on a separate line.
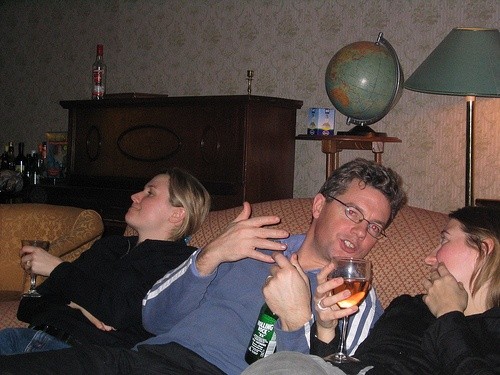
[0, 95, 303, 238]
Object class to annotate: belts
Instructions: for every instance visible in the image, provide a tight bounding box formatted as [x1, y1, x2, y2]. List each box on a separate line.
[28, 325, 82, 346]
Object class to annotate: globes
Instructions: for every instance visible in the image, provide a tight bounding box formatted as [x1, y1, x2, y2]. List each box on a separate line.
[325, 31, 404, 136]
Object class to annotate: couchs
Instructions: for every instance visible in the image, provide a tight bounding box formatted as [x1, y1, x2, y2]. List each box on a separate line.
[0, 203, 104, 328]
[123, 198, 450, 309]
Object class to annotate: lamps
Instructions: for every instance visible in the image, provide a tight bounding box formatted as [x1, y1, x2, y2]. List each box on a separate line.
[403, 27, 500, 206]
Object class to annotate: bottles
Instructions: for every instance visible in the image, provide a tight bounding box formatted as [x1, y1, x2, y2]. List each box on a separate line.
[0, 141, 59, 186]
[244, 303, 280, 365]
[92, 43, 108, 99]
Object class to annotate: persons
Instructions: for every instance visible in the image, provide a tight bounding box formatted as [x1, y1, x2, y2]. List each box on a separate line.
[0, 157, 407, 375]
[0, 168, 210, 356]
[239, 205, 500, 375]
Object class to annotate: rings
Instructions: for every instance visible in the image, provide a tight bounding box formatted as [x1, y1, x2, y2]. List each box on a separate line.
[27, 261, 30, 269]
[266, 275, 272, 283]
[318, 296, 327, 310]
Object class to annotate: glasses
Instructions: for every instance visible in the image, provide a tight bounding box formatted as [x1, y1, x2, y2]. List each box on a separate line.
[325, 193, 387, 240]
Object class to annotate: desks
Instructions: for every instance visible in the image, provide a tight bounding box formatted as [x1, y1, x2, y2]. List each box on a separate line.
[294, 134, 402, 180]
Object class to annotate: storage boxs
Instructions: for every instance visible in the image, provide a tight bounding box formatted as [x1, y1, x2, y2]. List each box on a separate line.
[308, 107, 336, 137]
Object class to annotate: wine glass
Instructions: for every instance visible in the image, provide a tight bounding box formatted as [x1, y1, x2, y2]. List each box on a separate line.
[20, 239, 51, 298]
[322, 256, 373, 363]
